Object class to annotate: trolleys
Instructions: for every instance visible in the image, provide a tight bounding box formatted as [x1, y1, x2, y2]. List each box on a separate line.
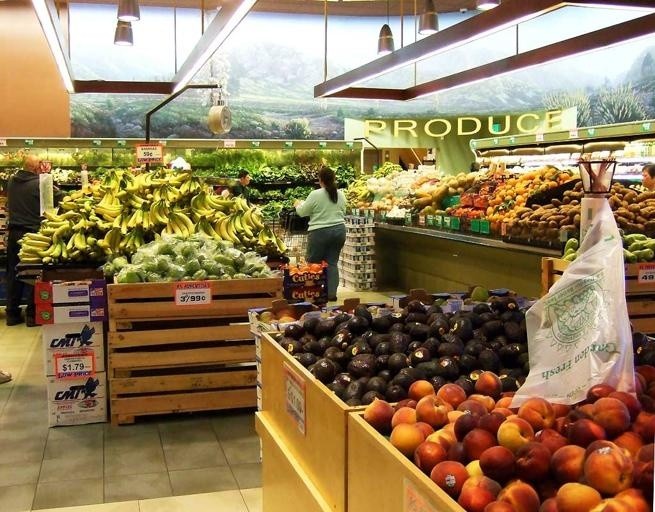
[272, 207, 310, 269]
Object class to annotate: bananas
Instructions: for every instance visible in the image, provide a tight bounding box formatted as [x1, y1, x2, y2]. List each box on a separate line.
[340, 161, 403, 215]
[16, 167, 290, 264]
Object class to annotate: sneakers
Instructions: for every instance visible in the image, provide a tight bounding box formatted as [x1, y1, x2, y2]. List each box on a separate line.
[6, 315, 44, 328]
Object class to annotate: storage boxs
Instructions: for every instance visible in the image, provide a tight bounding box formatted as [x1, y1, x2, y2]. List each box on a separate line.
[33, 279, 109, 428]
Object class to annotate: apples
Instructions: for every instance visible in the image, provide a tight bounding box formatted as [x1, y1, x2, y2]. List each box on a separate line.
[258, 311, 276, 325]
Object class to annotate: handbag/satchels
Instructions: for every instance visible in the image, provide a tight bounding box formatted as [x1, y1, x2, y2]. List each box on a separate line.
[279, 207, 309, 231]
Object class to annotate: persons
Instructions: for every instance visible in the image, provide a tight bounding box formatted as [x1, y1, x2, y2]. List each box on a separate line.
[292, 165, 350, 303]
[232, 170, 258, 204]
[641, 162, 655, 191]
[4, 150, 65, 329]
[0, 369, 15, 384]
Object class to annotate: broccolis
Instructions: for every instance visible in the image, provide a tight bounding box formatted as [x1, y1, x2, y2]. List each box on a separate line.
[103, 232, 272, 284]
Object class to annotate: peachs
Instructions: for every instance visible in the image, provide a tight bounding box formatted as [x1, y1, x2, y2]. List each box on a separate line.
[364, 365, 655, 512]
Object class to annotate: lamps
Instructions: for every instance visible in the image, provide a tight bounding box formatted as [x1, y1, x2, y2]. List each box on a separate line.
[377, 2, 397, 56]
[113, 1, 143, 51]
[417, 1, 440, 35]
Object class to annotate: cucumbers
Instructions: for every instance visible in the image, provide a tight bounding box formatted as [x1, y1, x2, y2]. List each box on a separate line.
[561, 232, 655, 263]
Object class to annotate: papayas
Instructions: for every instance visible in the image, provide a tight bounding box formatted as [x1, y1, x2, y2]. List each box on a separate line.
[409, 160, 506, 217]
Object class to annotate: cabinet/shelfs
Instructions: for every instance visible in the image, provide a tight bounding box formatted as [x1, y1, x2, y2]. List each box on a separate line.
[104, 274, 287, 427]
[538, 252, 653, 345]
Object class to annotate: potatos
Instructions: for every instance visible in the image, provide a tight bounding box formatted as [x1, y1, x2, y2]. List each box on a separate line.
[510, 180, 655, 245]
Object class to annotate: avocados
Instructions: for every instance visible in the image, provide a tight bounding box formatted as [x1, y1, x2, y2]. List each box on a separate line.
[274, 286, 532, 405]
[629, 320, 655, 365]
